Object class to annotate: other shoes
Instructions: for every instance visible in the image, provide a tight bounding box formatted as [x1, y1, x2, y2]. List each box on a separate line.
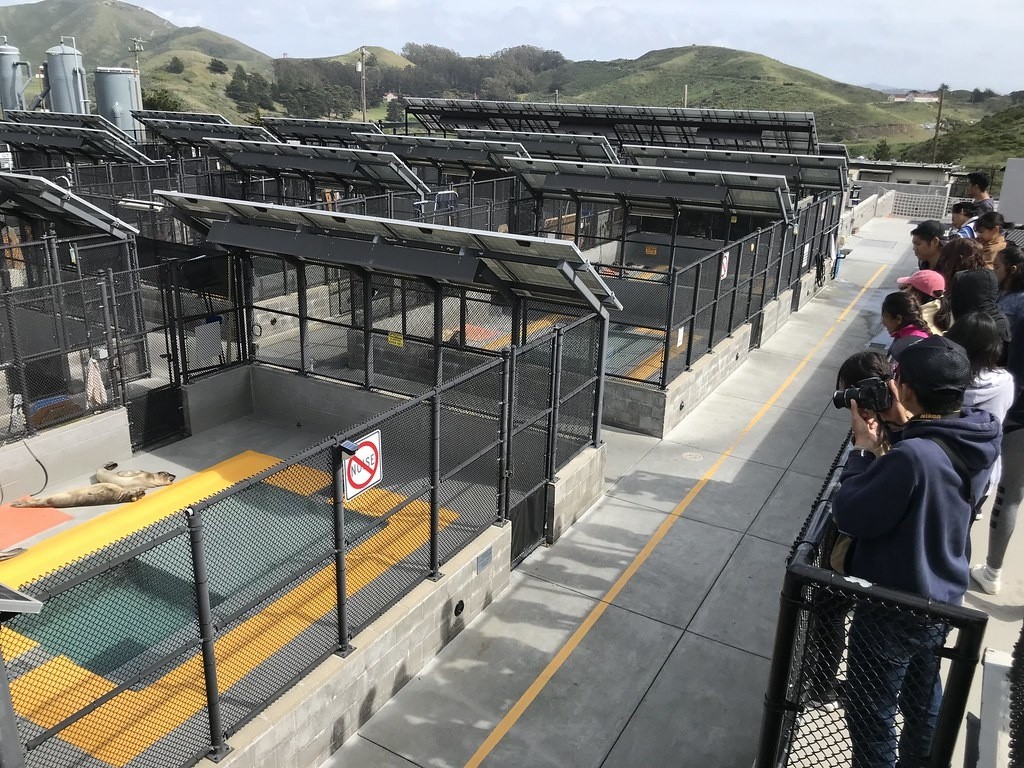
[814, 682, 848, 700]
[970, 563, 1000, 595]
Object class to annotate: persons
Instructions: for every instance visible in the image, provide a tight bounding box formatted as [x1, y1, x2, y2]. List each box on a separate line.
[949, 202, 978, 239]
[943, 239, 985, 296]
[911, 220, 949, 273]
[832, 334, 1003, 768]
[967, 171, 994, 216]
[974, 212, 1016, 269]
[808, 248, 1024, 711]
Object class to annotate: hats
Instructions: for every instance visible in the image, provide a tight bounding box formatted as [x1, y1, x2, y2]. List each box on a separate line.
[919, 220, 947, 239]
[889, 334, 970, 404]
[897, 270, 945, 298]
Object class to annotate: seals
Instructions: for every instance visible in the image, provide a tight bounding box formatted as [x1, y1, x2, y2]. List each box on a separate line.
[10, 483, 147, 508]
[96, 461, 177, 490]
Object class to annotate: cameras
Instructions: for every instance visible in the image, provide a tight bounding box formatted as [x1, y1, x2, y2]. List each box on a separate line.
[832, 377, 893, 413]
